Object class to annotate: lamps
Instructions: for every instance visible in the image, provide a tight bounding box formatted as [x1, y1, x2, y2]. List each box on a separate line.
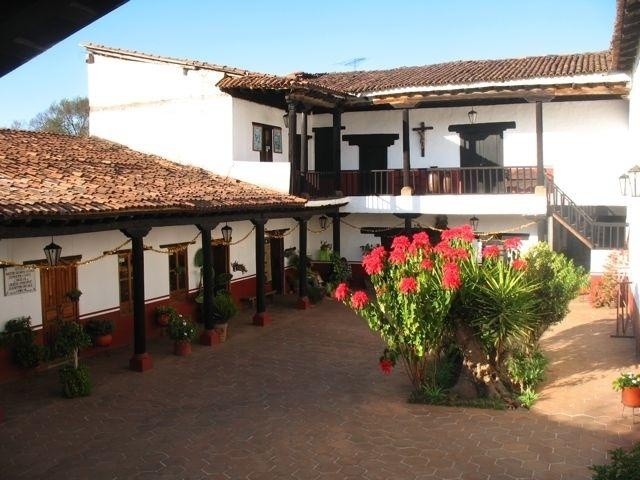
[319, 215, 327, 229]
[468, 106, 477, 124]
[628, 165, 640, 197]
[44, 236, 62, 269]
[620, 174, 629, 196]
[283, 111, 290, 129]
[221, 221, 232, 242]
[470, 216, 478, 231]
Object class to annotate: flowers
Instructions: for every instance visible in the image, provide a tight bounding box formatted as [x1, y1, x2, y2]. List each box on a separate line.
[612, 371, 640, 391]
[169, 314, 198, 340]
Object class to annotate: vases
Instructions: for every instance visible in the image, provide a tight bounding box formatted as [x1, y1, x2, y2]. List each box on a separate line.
[621, 386, 640, 407]
[174, 340, 191, 356]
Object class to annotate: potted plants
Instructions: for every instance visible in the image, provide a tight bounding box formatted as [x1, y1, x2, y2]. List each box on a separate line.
[212, 289, 241, 343]
[88, 318, 116, 345]
[157, 306, 176, 325]
